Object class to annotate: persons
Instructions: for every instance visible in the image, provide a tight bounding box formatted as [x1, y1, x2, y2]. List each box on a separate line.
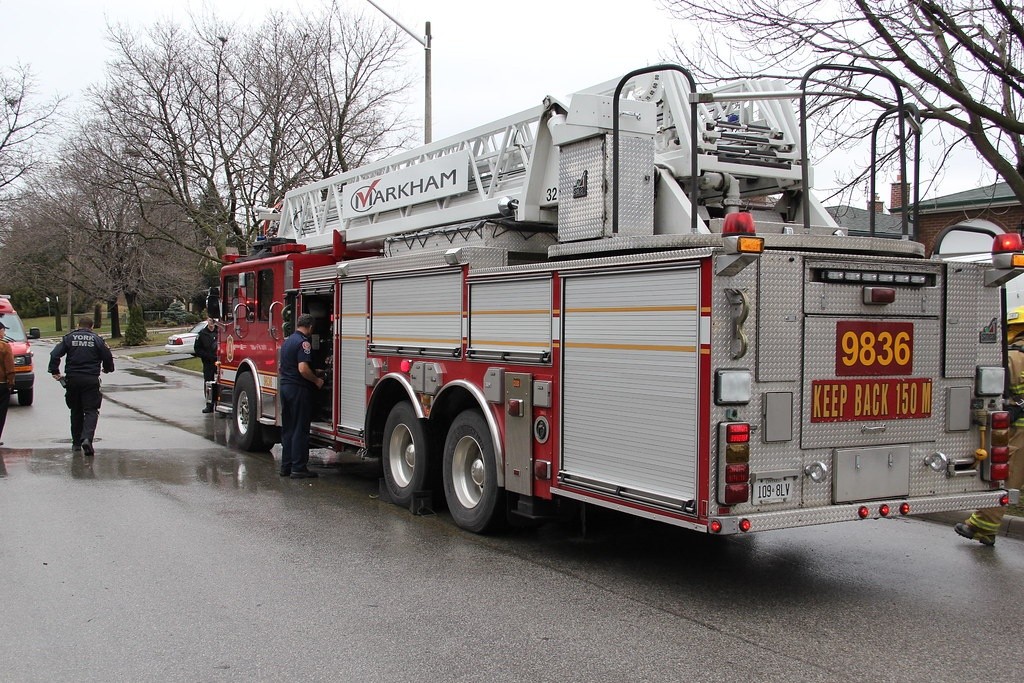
[0, 322, 16, 445]
[193, 316, 227, 413]
[954, 304, 1023, 547]
[279, 314, 325, 479]
[47, 316, 114, 457]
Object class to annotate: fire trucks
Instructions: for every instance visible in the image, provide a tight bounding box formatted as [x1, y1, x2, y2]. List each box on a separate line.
[201, 62, 1024, 537]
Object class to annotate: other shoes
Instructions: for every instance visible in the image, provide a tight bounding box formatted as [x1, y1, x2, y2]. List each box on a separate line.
[290, 467, 318, 479]
[73, 443, 82, 451]
[82, 438, 94, 455]
[202, 406, 213, 413]
[279, 469, 290, 476]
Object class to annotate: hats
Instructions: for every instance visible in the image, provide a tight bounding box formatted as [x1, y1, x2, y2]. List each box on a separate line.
[0, 321, 9, 330]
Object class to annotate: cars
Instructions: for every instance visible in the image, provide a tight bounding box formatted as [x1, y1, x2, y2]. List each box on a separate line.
[165, 318, 219, 357]
[0, 295, 41, 406]
